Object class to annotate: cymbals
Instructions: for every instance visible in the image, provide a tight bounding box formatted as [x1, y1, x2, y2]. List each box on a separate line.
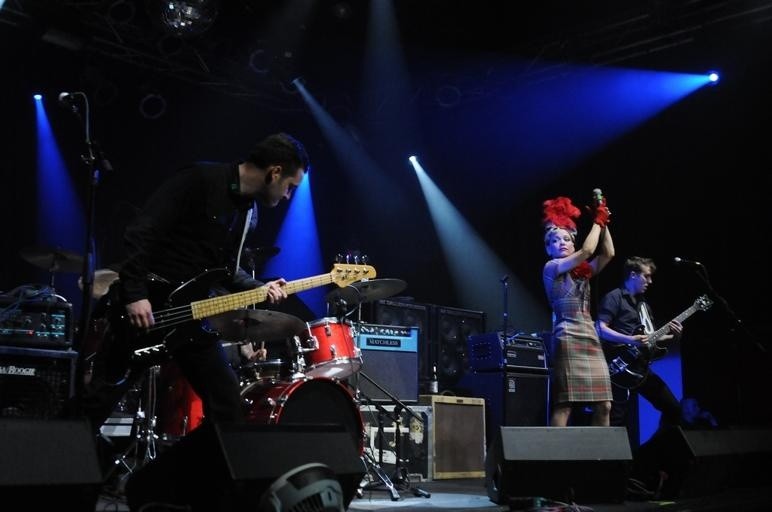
[205, 309, 305, 341]
[327, 279, 407, 306]
[15, 247, 85, 276]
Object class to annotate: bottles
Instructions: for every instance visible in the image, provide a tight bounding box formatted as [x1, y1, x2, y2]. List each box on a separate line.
[429, 363, 439, 394]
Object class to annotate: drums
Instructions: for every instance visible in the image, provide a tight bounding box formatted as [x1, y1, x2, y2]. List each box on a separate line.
[286, 316, 364, 383]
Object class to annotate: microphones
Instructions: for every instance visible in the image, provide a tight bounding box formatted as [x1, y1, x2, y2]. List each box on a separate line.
[674, 257, 701, 267]
[58, 92, 83, 104]
[593, 188, 603, 206]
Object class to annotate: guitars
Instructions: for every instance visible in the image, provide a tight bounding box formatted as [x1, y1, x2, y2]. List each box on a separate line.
[603, 294, 712, 392]
[99, 254, 377, 383]
[240, 377, 364, 459]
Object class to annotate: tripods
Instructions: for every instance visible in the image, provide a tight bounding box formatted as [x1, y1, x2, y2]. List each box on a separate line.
[360, 370, 431, 499]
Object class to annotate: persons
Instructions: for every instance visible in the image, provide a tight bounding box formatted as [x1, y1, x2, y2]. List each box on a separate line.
[541, 195, 614, 426]
[62, 132, 311, 426]
[595, 254, 684, 442]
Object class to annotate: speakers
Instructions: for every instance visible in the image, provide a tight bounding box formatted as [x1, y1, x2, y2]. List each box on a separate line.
[660, 424, 772, 502]
[484, 425, 634, 504]
[0, 419, 104, 511]
[473, 371, 552, 427]
[125, 419, 366, 512]
[431, 304, 487, 395]
[418, 394, 487, 480]
[361, 299, 430, 395]
[0, 345, 81, 421]
[354, 321, 420, 404]
